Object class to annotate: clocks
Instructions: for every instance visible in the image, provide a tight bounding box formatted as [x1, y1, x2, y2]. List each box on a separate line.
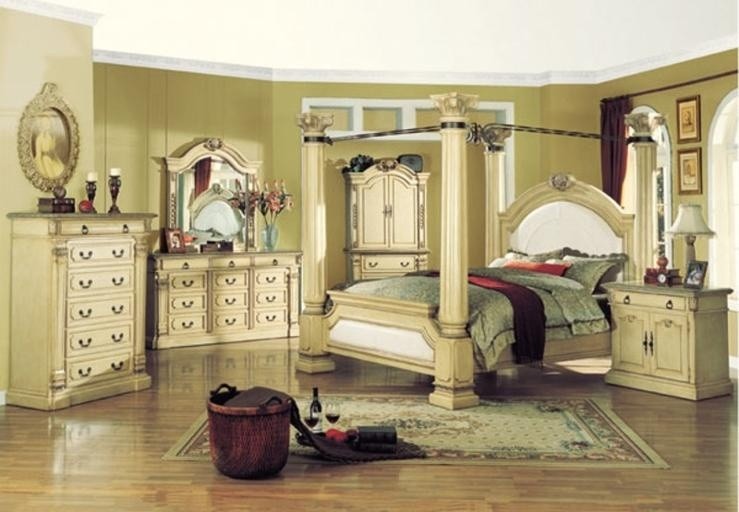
[657, 273, 670, 287]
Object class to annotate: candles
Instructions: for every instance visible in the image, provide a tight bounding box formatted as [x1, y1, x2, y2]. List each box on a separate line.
[110, 167, 120, 176]
[87, 173, 98, 182]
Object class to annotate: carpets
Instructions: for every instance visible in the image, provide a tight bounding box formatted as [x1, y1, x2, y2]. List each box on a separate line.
[160, 392, 670, 467]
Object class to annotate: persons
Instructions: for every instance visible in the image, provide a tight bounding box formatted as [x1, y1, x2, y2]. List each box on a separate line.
[34, 116, 66, 180]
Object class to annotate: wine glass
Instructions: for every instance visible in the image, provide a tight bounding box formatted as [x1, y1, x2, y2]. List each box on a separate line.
[325, 401, 340, 429]
[304, 406, 319, 430]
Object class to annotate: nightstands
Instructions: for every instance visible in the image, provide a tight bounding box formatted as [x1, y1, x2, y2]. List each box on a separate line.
[601, 281, 733, 400]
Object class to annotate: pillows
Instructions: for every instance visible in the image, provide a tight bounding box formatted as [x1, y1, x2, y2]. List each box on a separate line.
[488, 247, 628, 294]
[297, 93, 657, 411]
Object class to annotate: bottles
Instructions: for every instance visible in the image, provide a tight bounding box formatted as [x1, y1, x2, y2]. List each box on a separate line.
[309, 387, 321, 412]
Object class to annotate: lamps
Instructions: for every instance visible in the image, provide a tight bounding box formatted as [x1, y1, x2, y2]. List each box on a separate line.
[666, 205, 714, 269]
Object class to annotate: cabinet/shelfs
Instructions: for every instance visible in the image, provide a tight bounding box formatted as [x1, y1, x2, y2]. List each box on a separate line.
[344, 160, 431, 285]
[5, 214, 158, 412]
[151, 253, 302, 349]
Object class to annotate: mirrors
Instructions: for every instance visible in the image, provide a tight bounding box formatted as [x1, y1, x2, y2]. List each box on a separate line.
[166, 138, 264, 251]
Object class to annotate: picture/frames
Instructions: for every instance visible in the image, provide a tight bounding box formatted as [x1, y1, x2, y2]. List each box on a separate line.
[676, 95, 701, 144]
[165, 228, 186, 253]
[18, 82, 79, 191]
[677, 147, 702, 194]
[683, 261, 707, 288]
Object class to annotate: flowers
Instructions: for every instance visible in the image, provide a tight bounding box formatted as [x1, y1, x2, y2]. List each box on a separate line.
[227, 179, 257, 217]
[249, 177, 295, 246]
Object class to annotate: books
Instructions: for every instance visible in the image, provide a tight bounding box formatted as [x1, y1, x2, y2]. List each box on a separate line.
[358, 426, 397, 444]
[39, 205, 75, 213]
[643, 275, 684, 288]
[38, 198, 75, 205]
[646, 269, 681, 276]
[358, 443, 396, 453]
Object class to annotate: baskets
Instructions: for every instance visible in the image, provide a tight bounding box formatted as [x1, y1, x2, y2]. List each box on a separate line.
[206, 380, 292, 480]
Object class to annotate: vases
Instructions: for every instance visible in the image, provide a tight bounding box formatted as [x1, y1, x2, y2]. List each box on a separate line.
[262, 224, 280, 252]
[240, 215, 254, 247]
